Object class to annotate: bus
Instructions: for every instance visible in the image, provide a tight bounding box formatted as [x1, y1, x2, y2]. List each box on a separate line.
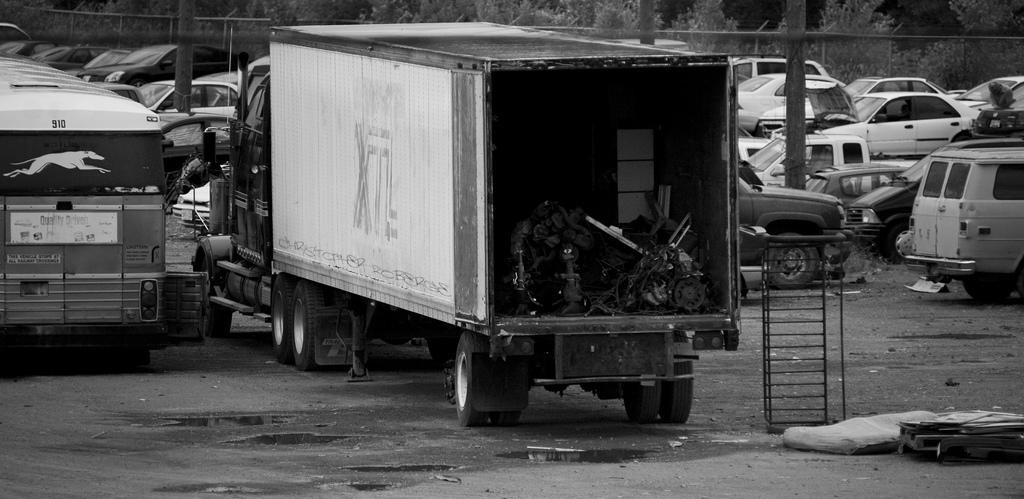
[0, 52, 167, 380]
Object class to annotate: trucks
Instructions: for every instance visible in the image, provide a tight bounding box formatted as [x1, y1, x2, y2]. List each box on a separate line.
[190, 19, 744, 429]
